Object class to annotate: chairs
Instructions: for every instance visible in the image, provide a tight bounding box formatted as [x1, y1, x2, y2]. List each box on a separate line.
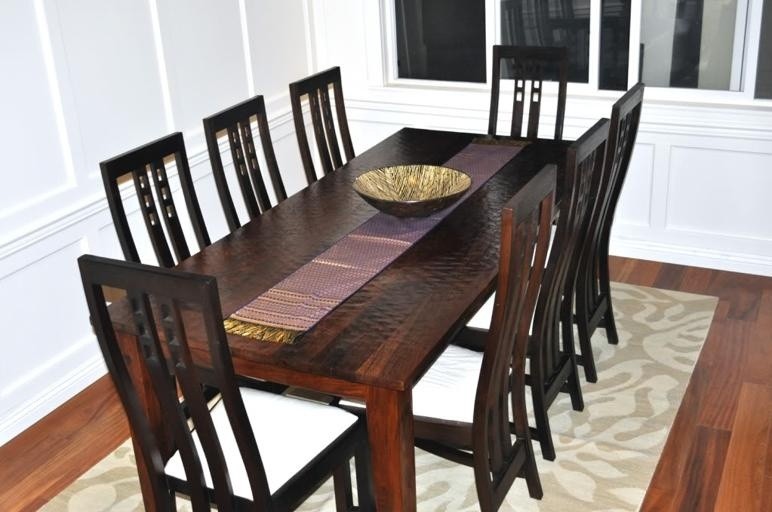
[460, 116, 610, 461]
[289, 65, 356, 185]
[529, 85, 646, 384]
[489, 46, 572, 140]
[340, 162, 557, 512]
[79, 255, 379, 512]
[203, 95, 286, 235]
[99, 132, 212, 272]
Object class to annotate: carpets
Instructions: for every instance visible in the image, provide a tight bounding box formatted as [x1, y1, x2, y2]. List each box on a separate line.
[35, 278, 718, 512]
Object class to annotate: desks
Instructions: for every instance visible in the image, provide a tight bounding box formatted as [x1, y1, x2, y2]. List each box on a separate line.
[88, 127, 579, 512]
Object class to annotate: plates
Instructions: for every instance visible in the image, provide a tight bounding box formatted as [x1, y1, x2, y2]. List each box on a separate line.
[352, 165, 472, 218]
